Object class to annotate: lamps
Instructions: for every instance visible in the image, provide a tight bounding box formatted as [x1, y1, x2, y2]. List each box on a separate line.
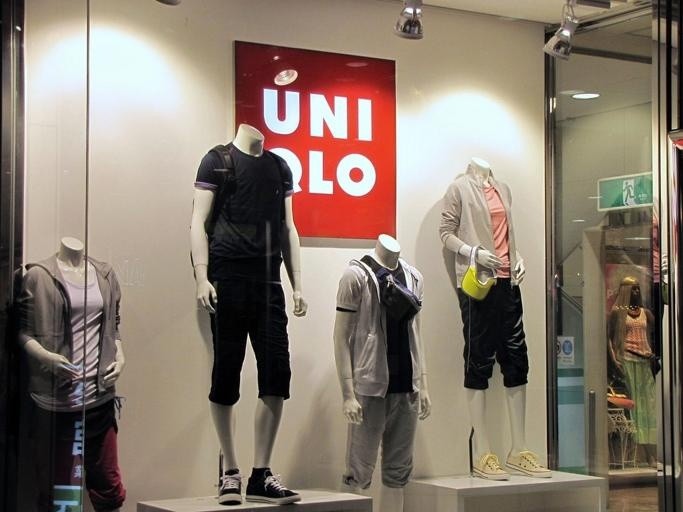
[393, 0, 424, 39]
[542, 0, 578, 61]
[271, 56, 299, 87]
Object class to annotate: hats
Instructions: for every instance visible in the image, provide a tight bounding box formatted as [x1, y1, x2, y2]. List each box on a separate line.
[461, 244, 498, 301]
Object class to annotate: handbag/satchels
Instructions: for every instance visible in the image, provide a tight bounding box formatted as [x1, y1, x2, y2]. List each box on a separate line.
[607, 393, 634, 408]
[649, 354, 661, 374]
[376, 267, 421, 322]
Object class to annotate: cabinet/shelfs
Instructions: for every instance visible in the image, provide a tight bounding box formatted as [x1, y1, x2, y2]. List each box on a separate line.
[402, 466, 607, 512]
[137, 486, 373, 512]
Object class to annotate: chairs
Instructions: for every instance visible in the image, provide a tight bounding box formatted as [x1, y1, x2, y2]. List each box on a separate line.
[606, 394, 637, 471]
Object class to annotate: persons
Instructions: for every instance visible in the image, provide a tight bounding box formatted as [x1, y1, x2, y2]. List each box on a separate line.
[16, 237, 126, 512]
[333, 233, 431, 512]
[190, 123, 309, 505]
[606, 276, 657, 467]
[438, 157, 552, 480]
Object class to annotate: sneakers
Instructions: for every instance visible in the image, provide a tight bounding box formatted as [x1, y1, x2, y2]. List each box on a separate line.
[472, 453, 509, 480]
[246, 470, 302, 506]
[504, 450, 552, 478]
[218, 473, 246, 504]
